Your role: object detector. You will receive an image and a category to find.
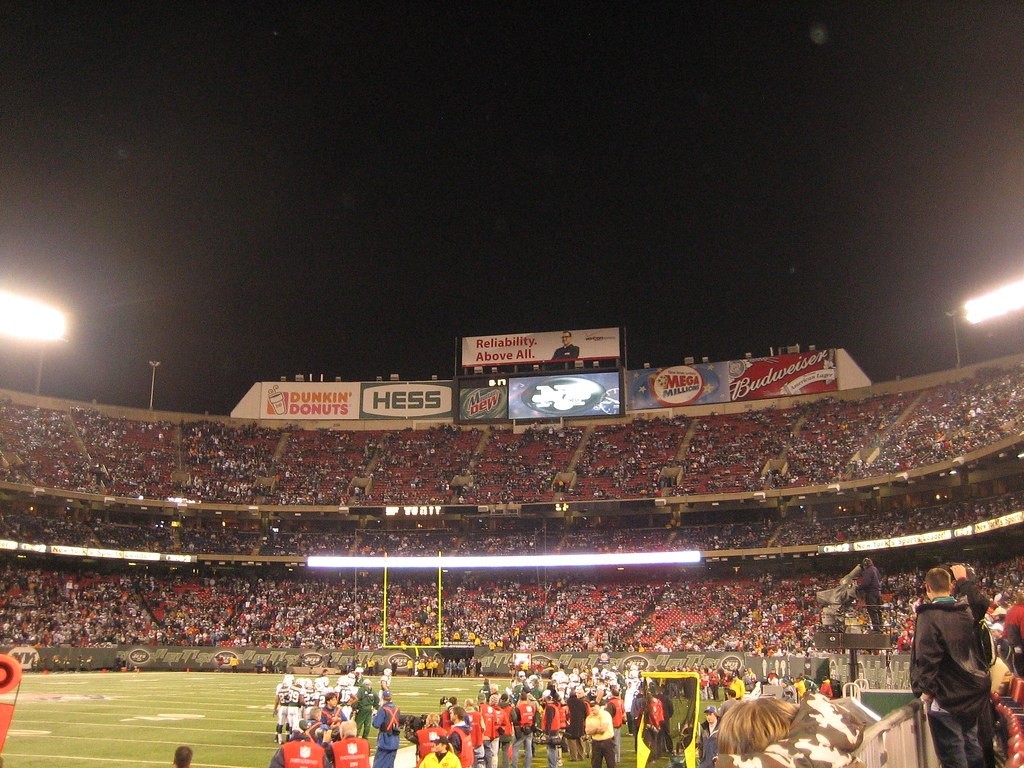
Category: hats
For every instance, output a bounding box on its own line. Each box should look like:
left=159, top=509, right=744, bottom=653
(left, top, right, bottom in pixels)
left=478, top=693, right=486, bottom=703
left=489, top=695, right=498, bottom=705
left=589, top=700, right=598, bottom=704
left=523, top=686, right=532, bottom=693
left=731, top=673, right=739, bottom=678
left=988, top=623, right=1004, bottom=632
left=382, top=690, right=391, bottom=697
left=743, top=668, right=748, bottom=672
left=429, top=735, right=450, bottom=743
left=990, top=606, right=1007, bottom=616
left=501, top=694, right=508, bottom=699
left=531, top=678, right=538, bottom=681
left=703, top=705, right=716, bottom=714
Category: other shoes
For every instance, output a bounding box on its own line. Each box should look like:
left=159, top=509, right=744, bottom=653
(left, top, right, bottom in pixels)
left=451, top=675, right=453, bottom=678
left=461, top=675, right=463, bottom=678
left=438, top=675, right=440, bottom=677
left=392, top=675, right=394, bottom=676
left=408, top=675, right=409, bottom=677
left=556, top=761, right=564, bottom=766
left=432, top=675, right=434, bottom=677
left=367, top=674, right=369, bottom=676
left=232, top=671, right=235, bottom=673
left=577, top=755, right=584, bottom=761
left=435, top=676, right=437, bottom=677
left=395, top=675, right=396, bottom=676
left=869, top=628, right=883, bottom=634
left=236, top=671, right=238, bottom=673
left=458, top=675, right=460, bottom=678
left=625, top=733, right=634, bottom=736
left=440, top=676, right=443, bottom=678
left=410, top=675, right=411, bottom=677
left=419, top=675, right=423, bottom=677
left=427, top=676, right=431, bottom=677
left=569, top=756, right=577, bottom=761
left=377, top=675, right=380, bottom=676
left=454, top=675, right=455, bottom=678
left=375, top=675, right=377, bottom=676
left=370, top=674, right=374, bottom=676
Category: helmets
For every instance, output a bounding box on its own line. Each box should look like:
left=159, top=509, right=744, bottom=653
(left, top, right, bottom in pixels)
left=336, top=676, right=349, bottom=685
left=518, top=671, right=526, bottom=680
left=630, top=665, right=639, bottom=671
left=295, top=678, right=305, bottom=687
left=569, top=674, right=580, bottom=685
left=551, top=672, right=569, bottom=683
left=526, top=675, right=537, bottom=690
left=383, top=668, right=392, bottom=677
left=321, top=677, right=329, bottom=686
left=543, top=690, right=551, bottom=696
left=355, top=667, right=363, bottom=672
left=609, top=678, right=617, bottom=686
left=314, top=678, right=323, bottom=683
left=607, top=672, right=616, bottom=679
left=304, top=678, right=312, bottom=688
left=315, top=683, right=324, bottom=691
left=283, top=675, right=294, bottom=685
left=557, top=691, right=564, bottom=700
left=629, top=671, right=639, bottom=678
left=555, top=684, right=571, bottom=699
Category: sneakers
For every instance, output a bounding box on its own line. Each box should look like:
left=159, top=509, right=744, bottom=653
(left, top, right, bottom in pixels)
left=274, top=735, right=278, bottom=742
left=278, top=735, right=284, bottom=744
left=286, top=734, right=289, bottom=741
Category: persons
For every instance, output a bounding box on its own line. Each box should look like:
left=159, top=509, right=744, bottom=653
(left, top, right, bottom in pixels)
left=552, top=331, right=580, bottom=369
left=0, top=359, right=1024, bottom=768
